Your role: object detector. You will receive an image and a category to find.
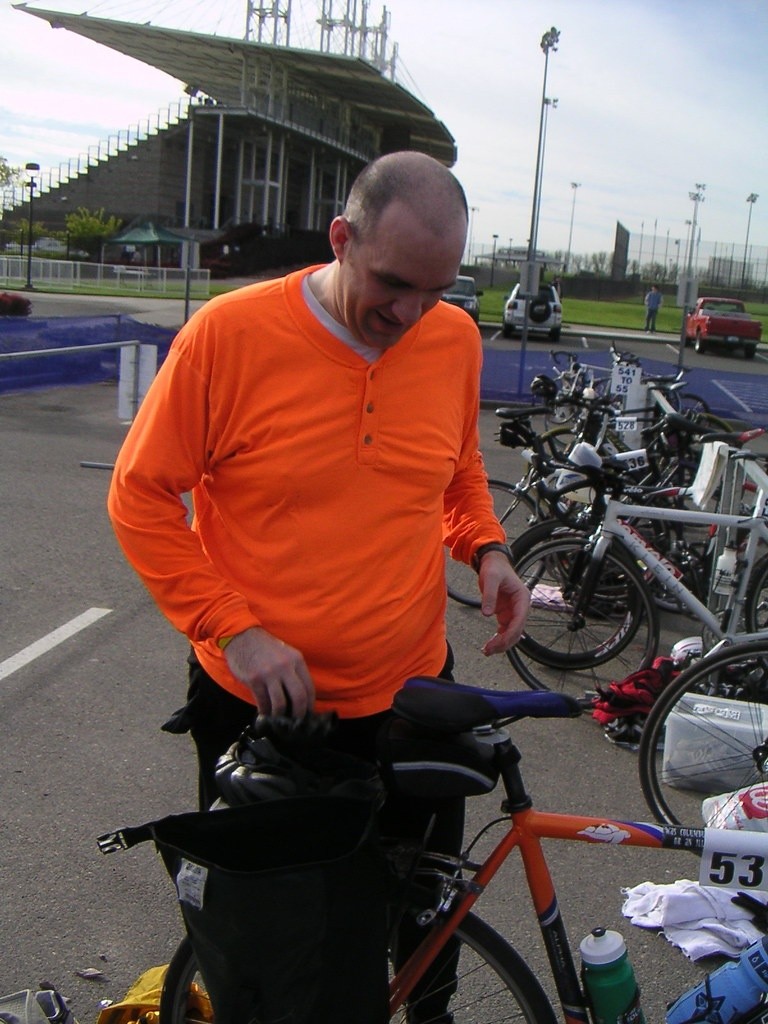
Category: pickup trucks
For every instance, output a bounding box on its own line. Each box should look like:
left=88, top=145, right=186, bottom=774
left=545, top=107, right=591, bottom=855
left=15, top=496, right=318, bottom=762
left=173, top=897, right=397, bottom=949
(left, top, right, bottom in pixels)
left=684, top=298, right=762, bottom=357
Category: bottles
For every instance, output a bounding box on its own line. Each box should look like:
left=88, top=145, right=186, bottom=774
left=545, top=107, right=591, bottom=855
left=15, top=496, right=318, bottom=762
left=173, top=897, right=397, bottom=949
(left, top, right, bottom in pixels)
left=712, top=540, right=737, bottom=596
left=580, top=926, right=648, bottom=1024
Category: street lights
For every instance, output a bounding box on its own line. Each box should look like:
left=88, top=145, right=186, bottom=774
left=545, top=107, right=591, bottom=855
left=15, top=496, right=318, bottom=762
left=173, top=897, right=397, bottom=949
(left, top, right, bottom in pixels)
left=489, top=233, right=499, bottom=289
left=740, top=191, right=760, bottom=289
left=562, top=182, right=582, bottom=271
left=674, top=183, right=707, bottom=280
left=25, top=163, right=43, bottom=289
left=517, top=22, right=560, bottom=397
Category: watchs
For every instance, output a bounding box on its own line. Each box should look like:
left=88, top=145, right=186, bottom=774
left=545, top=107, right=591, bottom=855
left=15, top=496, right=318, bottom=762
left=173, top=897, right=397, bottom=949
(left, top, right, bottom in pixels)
left=471, top=544, right=514, bottom=575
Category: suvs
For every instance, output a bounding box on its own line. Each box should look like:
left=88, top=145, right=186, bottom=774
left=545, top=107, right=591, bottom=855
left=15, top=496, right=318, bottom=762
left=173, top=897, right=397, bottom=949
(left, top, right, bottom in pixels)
left=502, top=282, right=564, bottom=336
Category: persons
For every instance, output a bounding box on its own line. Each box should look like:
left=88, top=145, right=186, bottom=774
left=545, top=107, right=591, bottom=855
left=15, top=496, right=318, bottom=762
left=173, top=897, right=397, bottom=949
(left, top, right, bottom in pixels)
left=105, top=151, right=532, bottom=1024
left=644, top=285, right=663, bottom=334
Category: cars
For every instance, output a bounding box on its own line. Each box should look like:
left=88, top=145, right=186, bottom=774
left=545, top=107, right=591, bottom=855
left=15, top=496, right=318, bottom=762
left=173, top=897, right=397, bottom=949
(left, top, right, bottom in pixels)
left=439, top=275, right=484, bottom=329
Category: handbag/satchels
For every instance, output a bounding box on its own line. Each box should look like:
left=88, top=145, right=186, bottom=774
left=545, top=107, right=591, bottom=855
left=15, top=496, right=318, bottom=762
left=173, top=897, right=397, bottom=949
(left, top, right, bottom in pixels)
left=94, top=794, right=391, bottom=1024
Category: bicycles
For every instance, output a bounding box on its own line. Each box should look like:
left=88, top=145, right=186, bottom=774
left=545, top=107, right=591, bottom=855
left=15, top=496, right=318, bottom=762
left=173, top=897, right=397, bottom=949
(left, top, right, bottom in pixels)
left=93, top=323, right=768, bottom=1024
